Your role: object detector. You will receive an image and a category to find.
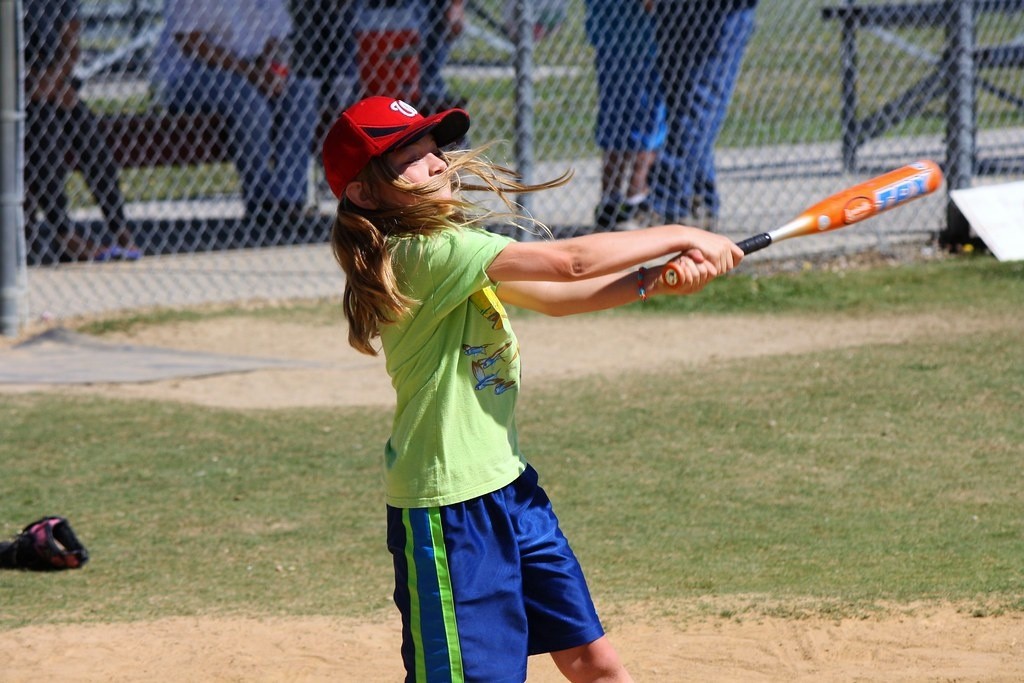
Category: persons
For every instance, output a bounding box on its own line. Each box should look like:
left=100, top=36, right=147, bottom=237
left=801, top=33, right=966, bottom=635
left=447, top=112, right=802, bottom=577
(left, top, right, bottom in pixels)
left=20, top=0, right=142, bottom=261
left=288, top=0, right=470, bottom=152
left=149, top=0, right=332, bottom=241
left=585, top=0, right=662, bottom=230
left=321, top=96, right=744, bottom=683
left=656, top=0, right=757, bottom=231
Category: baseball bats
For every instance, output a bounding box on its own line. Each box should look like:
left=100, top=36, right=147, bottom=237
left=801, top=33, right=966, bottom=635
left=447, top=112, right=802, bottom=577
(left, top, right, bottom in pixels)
left=662, top=159, right=943, bottom=288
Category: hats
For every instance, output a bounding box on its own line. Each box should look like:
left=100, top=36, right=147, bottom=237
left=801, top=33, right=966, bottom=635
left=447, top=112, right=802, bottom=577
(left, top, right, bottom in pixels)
left=321, top=94, right=471, bottom=199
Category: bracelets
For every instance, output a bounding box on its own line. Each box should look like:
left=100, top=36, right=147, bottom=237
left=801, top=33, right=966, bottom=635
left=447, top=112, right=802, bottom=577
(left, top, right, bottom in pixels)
left=638, top=266, right=651, bottom=302
